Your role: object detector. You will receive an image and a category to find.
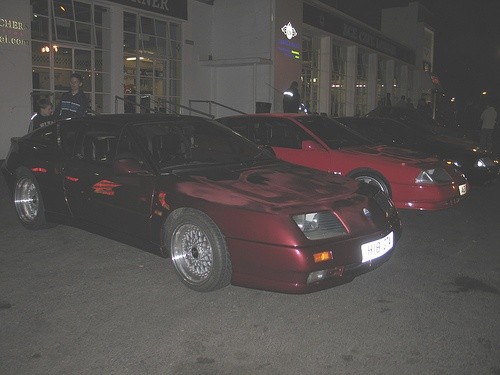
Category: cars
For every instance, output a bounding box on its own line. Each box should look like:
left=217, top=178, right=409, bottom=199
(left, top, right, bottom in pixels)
left=336, top=116, right=500, bottom=188
left=211, top=113, right=471, bottom=230
left=7, top=95, right=404, bottom=292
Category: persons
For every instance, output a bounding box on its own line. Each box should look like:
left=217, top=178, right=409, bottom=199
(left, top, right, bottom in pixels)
left=283, top=80, right=300, bottom=112
left=478, top=99, right=497, bottom=149
left=385, top=92, right=414, bottom=107
left=55, top=73, right=88, bottom=119
left=27, top=97, right=59, bottom=134
